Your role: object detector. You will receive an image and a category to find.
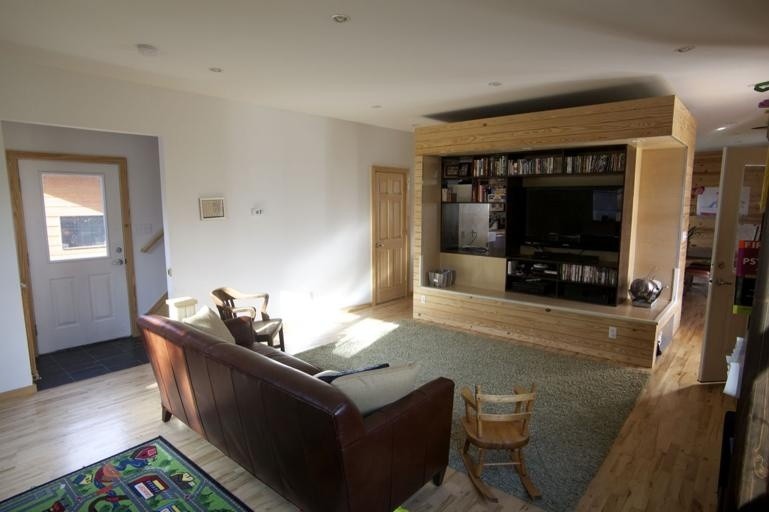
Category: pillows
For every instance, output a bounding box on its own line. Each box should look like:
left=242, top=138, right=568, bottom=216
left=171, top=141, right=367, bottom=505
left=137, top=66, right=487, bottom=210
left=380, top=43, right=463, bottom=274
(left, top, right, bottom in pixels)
left=175, top=301, right=256, bottom=350
left=313, top=358, right=422, bottom=414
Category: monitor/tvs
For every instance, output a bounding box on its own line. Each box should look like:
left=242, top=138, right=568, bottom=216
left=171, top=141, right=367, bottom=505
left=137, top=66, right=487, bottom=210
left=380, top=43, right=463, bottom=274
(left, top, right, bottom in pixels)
left=525, top=184, right=624, bottom=252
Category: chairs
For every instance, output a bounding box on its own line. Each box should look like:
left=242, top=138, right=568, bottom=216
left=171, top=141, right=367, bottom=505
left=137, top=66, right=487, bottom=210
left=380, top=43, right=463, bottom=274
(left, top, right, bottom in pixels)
left=211, top=286, right=285, bottom=353
left=459, top=381, right=545, bottom=502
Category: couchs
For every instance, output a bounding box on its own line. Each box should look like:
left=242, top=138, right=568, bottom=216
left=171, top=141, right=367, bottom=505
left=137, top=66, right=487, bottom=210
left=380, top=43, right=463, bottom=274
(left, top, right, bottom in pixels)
left=137, top=309, right=456, bottom=512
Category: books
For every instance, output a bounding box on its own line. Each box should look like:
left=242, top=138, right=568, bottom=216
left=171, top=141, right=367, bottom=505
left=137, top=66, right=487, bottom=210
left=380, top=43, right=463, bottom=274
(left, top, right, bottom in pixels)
left=471, top=151, right=625, bottom=203
left=560, top=264, right=618, bottom=287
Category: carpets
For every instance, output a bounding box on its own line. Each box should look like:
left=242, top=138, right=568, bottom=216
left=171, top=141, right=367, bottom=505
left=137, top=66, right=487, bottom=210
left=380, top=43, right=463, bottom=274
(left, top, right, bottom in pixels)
left=288, top=315, right=651, bottom=512
left=0, top=433, right=255, bottom=512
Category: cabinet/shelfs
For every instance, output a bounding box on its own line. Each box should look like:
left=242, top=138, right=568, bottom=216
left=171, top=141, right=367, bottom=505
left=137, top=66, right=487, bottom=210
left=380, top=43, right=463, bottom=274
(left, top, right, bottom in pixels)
left=437, top=145, right=642, bottom=309
